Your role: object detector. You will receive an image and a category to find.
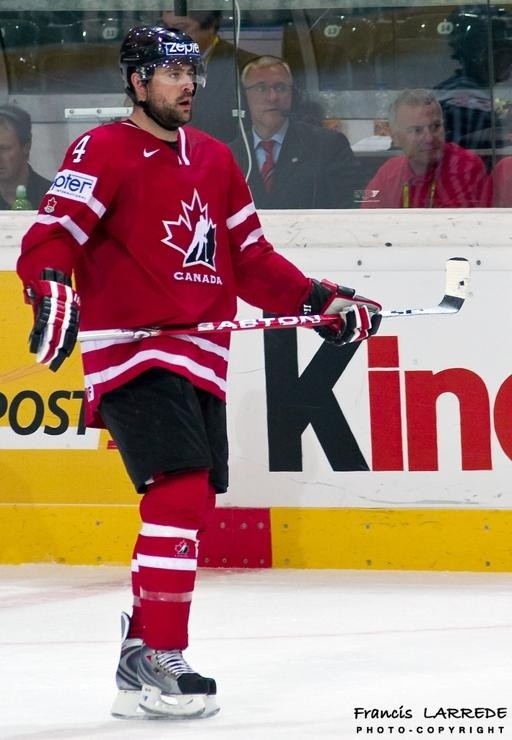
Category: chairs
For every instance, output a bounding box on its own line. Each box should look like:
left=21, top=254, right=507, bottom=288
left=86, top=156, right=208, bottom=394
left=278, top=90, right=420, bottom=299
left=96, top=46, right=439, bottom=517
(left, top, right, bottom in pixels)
left=478, top=10, right=512, bottom=88
left=282, top=15, right=396, bottom=89
left=42, top=23, right=81, bottom=44
left=0, top=19, right=40, bottom=47
left=393, top=9, right=483, bottom=90
left=79, top=18, right=143, bottom=42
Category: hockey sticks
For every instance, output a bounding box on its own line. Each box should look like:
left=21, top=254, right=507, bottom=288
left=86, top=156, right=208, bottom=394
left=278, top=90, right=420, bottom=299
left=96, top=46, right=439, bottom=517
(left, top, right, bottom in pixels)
left=76, top=256, right=472, bottom=344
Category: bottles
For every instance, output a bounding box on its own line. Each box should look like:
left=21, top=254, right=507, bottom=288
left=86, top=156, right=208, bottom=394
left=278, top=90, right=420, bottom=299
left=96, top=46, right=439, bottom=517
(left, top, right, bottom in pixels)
left=10, top=184, right=33, bottom=211
left=374, top=82, right=393, bottom=137
left=321, top=85, right=340, bottom=131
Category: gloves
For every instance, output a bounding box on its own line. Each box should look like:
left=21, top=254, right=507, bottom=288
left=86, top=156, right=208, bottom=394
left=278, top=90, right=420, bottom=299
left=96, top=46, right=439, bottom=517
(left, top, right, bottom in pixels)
left=301, top=279, right=383, bottom=345
left=27, top=269, right=81, bottom=372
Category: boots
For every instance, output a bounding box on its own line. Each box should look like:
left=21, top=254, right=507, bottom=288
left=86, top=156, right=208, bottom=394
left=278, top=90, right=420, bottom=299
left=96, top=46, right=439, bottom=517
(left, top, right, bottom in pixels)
left=115, top=612, right=216, bottom=694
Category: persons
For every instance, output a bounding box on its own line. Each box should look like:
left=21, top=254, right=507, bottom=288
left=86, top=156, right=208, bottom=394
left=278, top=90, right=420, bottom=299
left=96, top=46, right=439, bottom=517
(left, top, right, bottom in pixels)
left=0, top=105, right=54, bottom=213
left=355, top=88, right=486, bottom=207
left=13, top=20, right=383, bottom=700
left=158, top=8, right=262, bottom=150
left=227, top=55, right=359, bottom=207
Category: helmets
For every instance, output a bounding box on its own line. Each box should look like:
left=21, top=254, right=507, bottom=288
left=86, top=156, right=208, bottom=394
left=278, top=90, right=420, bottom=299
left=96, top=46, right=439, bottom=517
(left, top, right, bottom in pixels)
left=120, top=24, right=201, bottom=89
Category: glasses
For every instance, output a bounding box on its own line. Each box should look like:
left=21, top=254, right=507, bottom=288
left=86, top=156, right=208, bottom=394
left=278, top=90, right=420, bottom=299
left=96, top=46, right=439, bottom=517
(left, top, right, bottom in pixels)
left=245, top=83, right=288, bottom=95
